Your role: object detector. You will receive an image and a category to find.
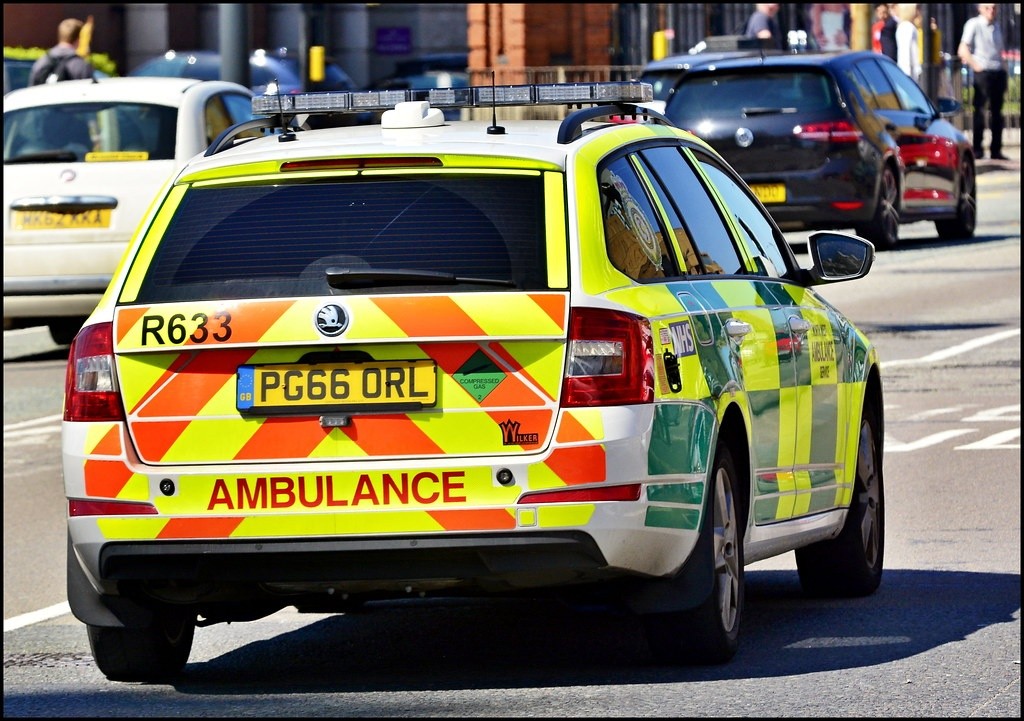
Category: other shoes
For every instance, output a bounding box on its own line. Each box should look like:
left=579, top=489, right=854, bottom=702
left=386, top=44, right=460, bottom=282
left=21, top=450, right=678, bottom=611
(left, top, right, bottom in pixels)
left=991, top=155, right=1007, bottom=159
left=974, top=155, right=981, bottom=159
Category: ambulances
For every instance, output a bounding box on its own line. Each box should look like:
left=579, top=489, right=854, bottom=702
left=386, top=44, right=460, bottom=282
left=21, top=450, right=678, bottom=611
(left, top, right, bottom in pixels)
left=60, top=81, right=885, bottom=680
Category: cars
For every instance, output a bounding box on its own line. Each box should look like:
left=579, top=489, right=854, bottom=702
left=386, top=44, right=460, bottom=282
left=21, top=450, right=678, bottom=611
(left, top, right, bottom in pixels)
left=0, top=39, right=471, bottom=346
left=631, top=49, right=977, bottom=247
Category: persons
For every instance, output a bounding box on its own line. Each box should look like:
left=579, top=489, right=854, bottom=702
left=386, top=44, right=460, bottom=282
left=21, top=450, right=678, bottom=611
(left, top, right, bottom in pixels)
left=27, top=18, right=91, bottom=87
left=957, top=2, right=1024, bottom=160
left=809, top=3, right=942, bottom=96
left=743, top=3, right=784, bottom=55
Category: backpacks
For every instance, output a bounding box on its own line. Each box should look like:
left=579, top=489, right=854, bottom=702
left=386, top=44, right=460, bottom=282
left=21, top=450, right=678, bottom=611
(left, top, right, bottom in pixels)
left=33, top=50, right=78, bottom=85
